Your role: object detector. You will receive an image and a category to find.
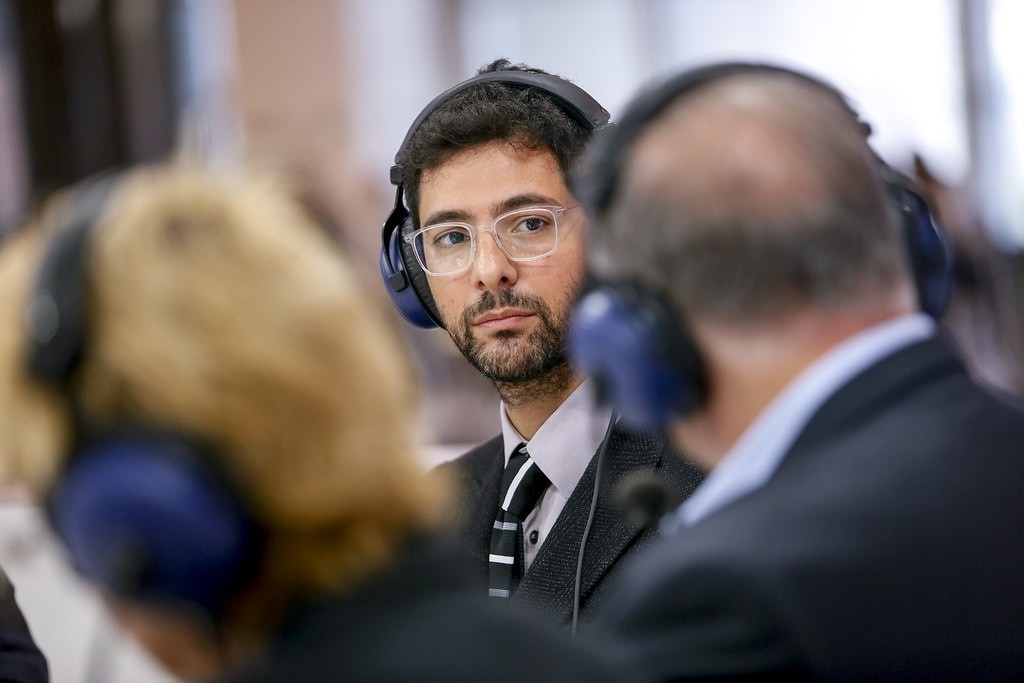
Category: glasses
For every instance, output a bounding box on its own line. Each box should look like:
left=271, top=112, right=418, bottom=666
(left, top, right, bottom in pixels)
left=403, top=201, right=580, bottom=276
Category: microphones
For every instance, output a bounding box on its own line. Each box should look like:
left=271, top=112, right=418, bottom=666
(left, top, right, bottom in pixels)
left=618, top=441, right=671, bottom=513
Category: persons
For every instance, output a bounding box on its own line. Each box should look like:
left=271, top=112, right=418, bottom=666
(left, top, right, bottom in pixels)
left=381, top=58, right=703, bottom=633
left=0, top=165, right=618, bottom=682
left=570, top=59, right=1023, bottom=683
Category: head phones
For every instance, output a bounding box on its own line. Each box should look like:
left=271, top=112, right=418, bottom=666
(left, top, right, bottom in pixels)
left=28, top=167, right=268, bottom=620
left=379, top=71, right=608, bottom=332
left=564, top=60, right=958, bottom=433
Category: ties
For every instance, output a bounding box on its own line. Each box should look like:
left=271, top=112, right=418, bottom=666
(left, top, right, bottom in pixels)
left=486, top=451, right=549, bottom=607
